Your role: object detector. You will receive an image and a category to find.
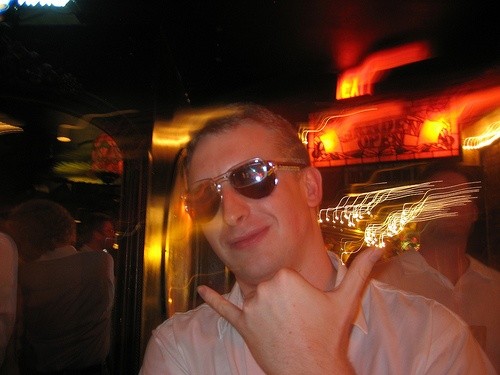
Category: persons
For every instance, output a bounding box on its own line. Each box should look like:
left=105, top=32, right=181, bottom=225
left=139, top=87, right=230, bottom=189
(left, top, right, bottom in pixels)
left=364, top=169, right=500, bottom=374
left=138, top=104, right=497, bottom=375
left=80, top=212, right=115, bottom=256
left=19, top=198, right=115, bottom=375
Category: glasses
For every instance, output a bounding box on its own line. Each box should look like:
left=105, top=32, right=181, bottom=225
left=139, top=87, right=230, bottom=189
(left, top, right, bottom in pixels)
left=181, top=158, right=312, bottom=224
